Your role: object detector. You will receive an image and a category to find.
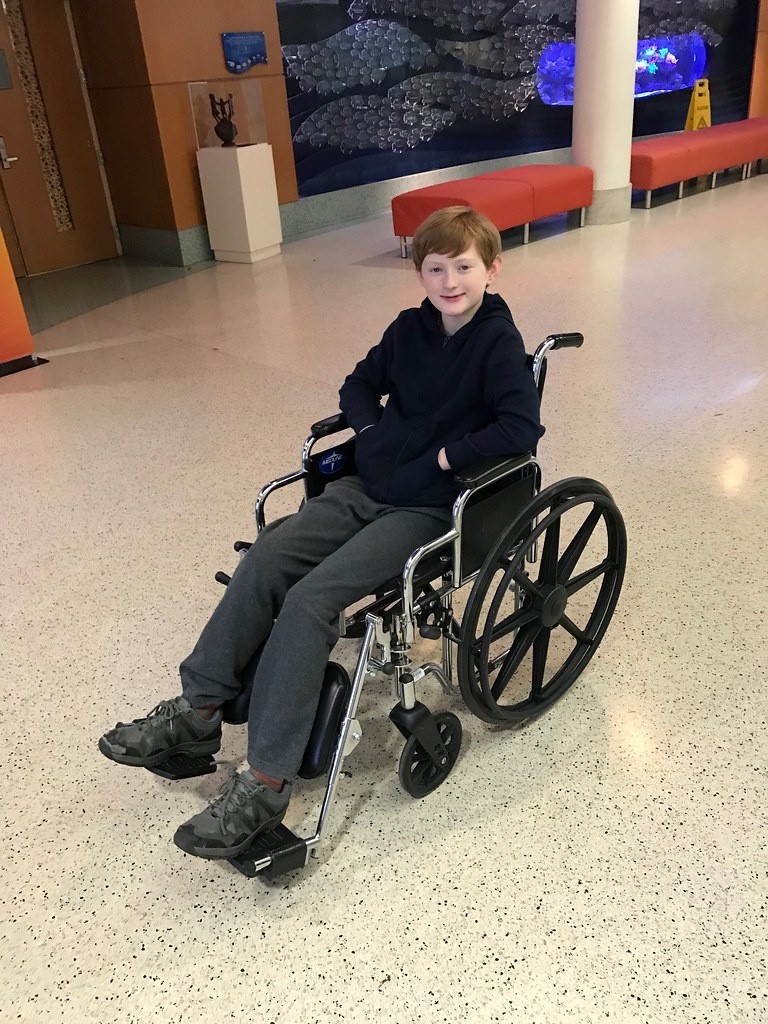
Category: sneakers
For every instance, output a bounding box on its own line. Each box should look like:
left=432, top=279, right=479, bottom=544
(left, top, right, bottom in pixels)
left=98, top=696, right=223, bottom=767
left=174, top=760, right=294, bottom=861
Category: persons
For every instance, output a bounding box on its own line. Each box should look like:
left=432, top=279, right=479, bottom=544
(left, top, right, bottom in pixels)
left=95, top=201, right=547, bottom=862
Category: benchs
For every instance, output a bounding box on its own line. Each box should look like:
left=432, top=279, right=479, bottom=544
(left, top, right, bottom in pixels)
left=739, top=117, right=768, bottom=178
left=391, top=163, right=593, bottom=259
left=631, top=122, right=756, bottom=209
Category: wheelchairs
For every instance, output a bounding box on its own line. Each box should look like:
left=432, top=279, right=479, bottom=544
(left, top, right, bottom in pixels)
left=146, top=328, right=628, bottom=883
